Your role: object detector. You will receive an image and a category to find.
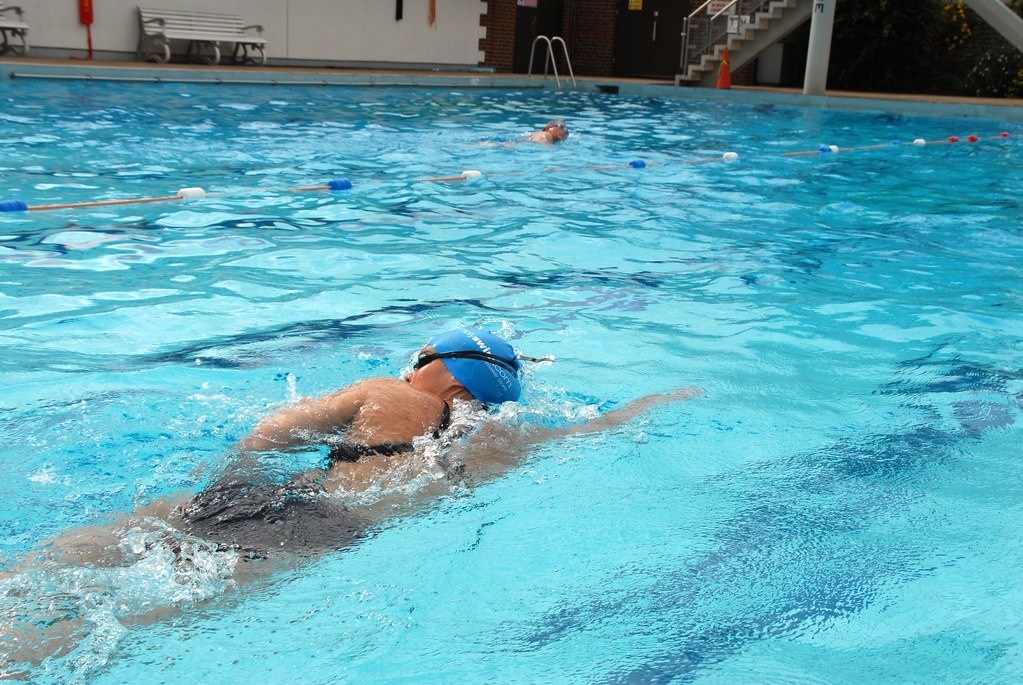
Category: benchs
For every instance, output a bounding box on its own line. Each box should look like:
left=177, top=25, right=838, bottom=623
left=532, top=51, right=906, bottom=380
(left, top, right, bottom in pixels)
left=137, top=4, right=269, bottom=66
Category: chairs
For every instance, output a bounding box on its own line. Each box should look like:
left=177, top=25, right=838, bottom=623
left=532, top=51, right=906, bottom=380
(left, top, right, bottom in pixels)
left=0, top=2, right=32, bottom=58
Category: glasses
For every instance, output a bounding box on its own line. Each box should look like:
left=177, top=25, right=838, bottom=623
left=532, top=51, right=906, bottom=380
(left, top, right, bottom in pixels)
left=413, top=351, right=521, bottom=375
left=549, top=123, right=569, bottom=136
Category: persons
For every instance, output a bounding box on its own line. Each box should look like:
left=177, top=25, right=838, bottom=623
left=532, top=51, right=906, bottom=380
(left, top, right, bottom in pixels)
left=480, top=121, right=570, bottom=152
left=2, top=323, right=701, bottom=672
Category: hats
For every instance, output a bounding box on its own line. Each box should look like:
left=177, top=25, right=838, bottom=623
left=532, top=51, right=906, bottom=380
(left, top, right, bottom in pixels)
left=425, top=329, right=520, bottom=410
